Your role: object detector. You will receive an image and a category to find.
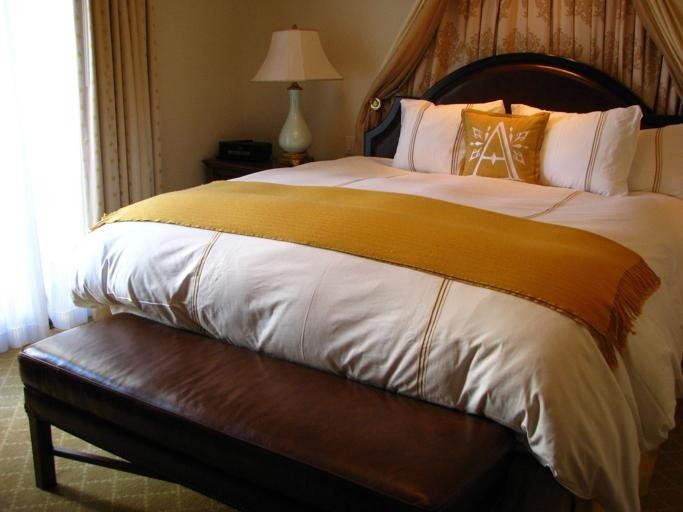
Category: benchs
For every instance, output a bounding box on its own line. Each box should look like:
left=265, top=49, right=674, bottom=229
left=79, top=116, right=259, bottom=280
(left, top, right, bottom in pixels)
left=19, top=313, right=526, bottom=512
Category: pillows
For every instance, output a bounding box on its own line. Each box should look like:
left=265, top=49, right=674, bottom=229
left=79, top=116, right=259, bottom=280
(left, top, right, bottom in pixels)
left=391, top=95, right=681, bottom=198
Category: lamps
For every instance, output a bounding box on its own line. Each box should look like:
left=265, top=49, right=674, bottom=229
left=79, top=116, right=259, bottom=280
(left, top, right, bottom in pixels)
left=249, top=22, right=344, bottom=167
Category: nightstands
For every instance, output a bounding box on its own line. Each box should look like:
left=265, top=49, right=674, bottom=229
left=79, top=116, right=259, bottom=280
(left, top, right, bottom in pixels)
left=210, top=158, right=276, bottom=180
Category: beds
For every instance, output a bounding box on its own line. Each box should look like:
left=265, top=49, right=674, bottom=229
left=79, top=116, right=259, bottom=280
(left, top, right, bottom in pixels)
left=98, top=51, right=682, bottom=511
